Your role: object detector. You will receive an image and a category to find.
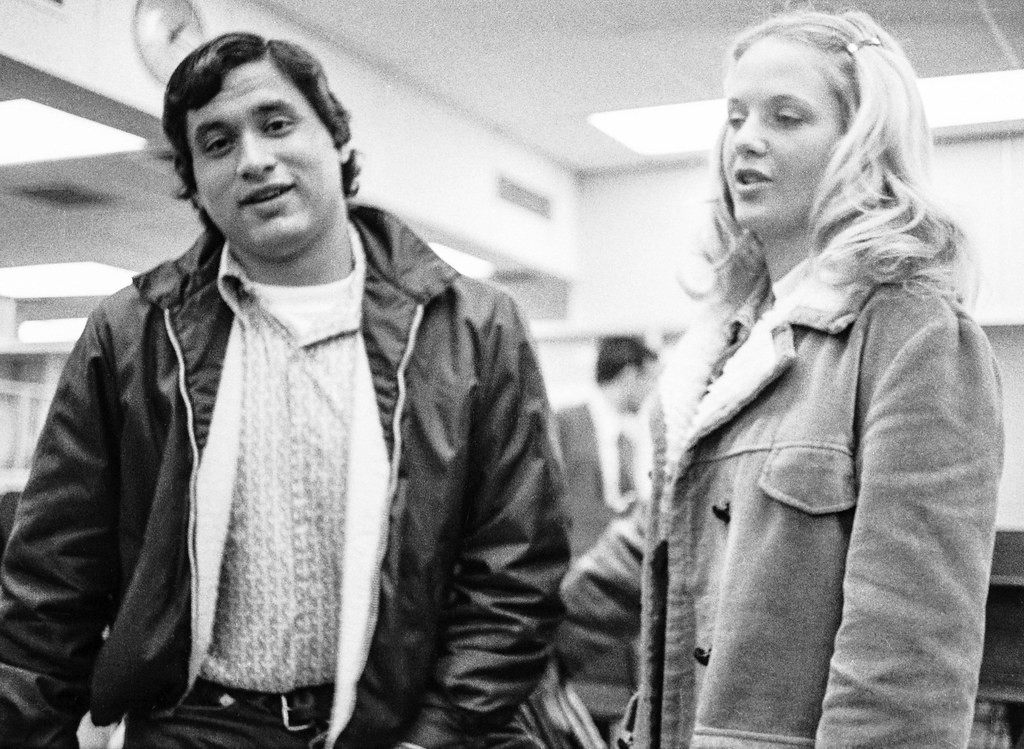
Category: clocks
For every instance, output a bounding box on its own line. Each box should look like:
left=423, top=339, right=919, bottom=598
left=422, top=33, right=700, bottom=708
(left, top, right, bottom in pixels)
left=96, top=0, right=217, bottom=102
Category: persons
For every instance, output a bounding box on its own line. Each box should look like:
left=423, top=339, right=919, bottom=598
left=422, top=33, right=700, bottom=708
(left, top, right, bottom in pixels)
left=556, top=332, right=660, bottom=748
left=0, top=32, right=572, bottom=749
left=529, top=7, right=1008, bottom=749
